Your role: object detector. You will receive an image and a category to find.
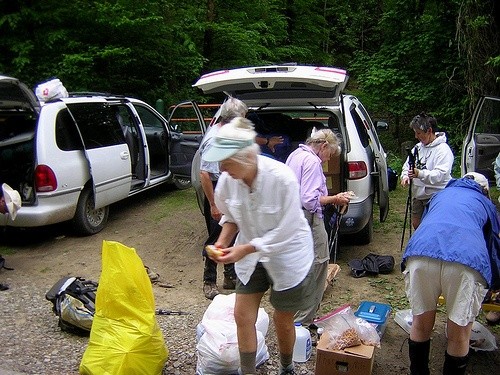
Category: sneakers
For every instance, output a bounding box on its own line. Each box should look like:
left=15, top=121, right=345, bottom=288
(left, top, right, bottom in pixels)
left=223, top=277, right=237, bottom=289
left=203, top=280, right=219, bottom=299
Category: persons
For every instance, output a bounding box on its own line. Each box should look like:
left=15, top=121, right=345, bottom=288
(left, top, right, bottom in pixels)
left=199, top=97, right=283, bottom=299
left=0, top=183, right=21, bottom=290
left=402, top=112, right=454, bottom=229
left=204, top=118, right=315, bottom=375
left=400, top=171, right=500, bottom=375
left=285, top=128, right=349, bottom=346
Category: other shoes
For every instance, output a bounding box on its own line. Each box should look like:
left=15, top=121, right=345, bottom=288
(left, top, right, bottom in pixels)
left=278, top=362, right=295, bottom=375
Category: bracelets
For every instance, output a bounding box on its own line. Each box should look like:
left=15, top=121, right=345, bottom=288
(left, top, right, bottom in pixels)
left=265, top=138, right=270, bottom=145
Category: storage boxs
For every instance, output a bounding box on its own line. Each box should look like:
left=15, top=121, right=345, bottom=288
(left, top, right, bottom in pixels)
left=324, top=174, right=341, bottom=194
left=355, top=301, right=391, bottom=340
left=315, top=334, right=374, bottom=375
left=322, top=154, right=341, bottom=173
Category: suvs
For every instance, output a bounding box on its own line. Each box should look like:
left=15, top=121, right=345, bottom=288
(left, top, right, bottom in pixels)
left=191, top=63, right=391, bottom=246
left=0, top=75, right=207, bottom=237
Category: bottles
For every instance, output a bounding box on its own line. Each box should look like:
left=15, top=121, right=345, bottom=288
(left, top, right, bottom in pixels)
left=292, top=322, right=312, bottom=363
left=317, top=327, right=323, bottom=347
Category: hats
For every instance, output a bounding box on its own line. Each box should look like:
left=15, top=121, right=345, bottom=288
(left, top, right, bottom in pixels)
left=202, top=137, right=253, bottom=161
left=465, top=171, right=491, bottom=199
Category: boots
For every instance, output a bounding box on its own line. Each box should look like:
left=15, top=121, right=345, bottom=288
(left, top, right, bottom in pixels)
left=406, top=335, right=429, bottom=375
left=444, top=351, right=471, bottom=375
